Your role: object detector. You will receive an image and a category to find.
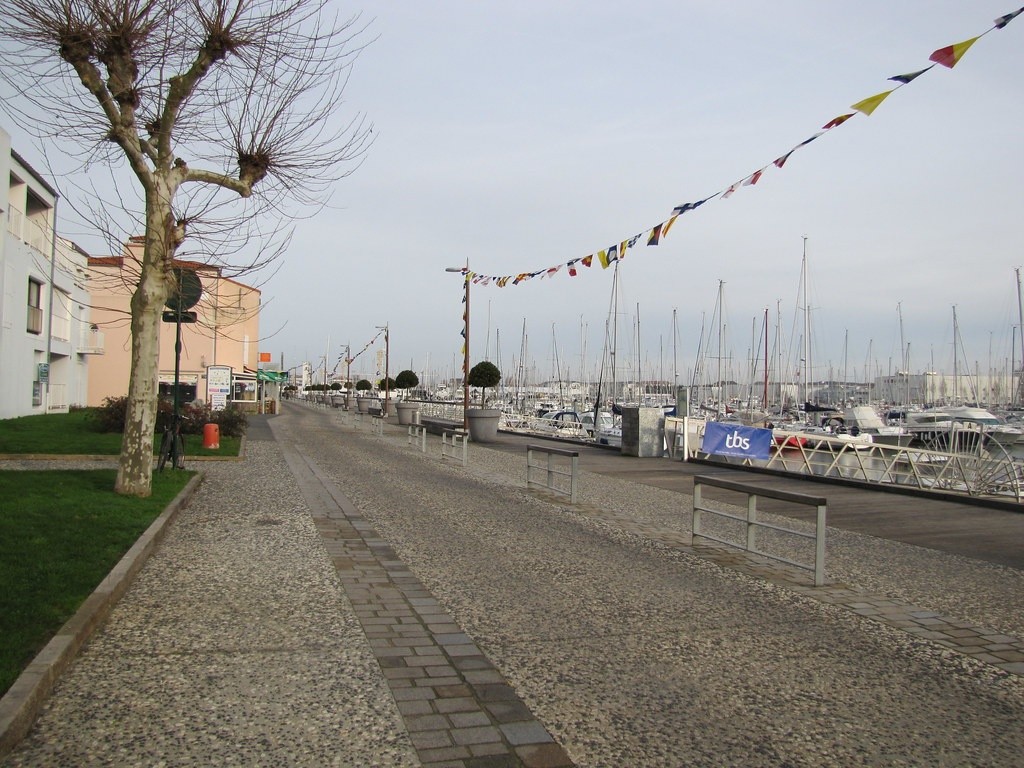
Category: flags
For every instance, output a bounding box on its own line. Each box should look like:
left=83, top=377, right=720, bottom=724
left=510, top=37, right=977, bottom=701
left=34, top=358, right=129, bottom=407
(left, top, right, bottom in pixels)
left=461, top=266, right=470, bottom=389
left=662, top=215, right=679, bottom=238
left=626, top=235, right=642, bottom=248
left=669, top=4, right=1024, bottom=216
left=647, top=223, right=664, bottom=246
left=620, top=240, right=628, bottom=258
left=596, top=250, right=610, bottom=270
left=470, top=253, right=581, bottom=288
left=606, top=245, right=618, bottom=264
left=581, top=255, right=593, bottom=268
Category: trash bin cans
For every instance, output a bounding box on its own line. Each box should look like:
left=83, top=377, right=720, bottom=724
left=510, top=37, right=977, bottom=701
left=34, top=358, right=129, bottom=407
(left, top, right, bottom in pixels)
left=202, top=422, right=221, bottom=450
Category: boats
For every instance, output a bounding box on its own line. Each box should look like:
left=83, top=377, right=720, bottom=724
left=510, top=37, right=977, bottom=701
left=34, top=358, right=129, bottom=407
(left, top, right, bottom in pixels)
left=377, top=236, right=1023, bottom=451
